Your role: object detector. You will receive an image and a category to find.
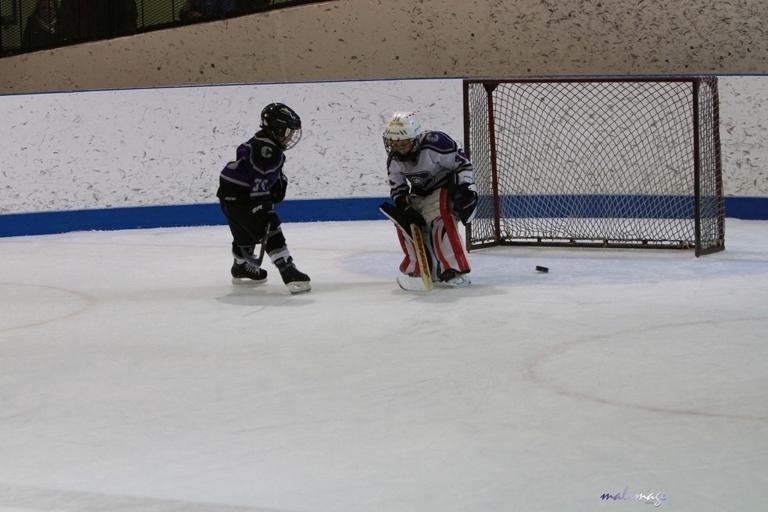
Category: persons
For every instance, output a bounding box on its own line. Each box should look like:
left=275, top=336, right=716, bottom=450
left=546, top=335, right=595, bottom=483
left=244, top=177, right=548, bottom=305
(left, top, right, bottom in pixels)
left=216, top=102, right=311, bottom=286
left=380, top=110, right=479, bottom=283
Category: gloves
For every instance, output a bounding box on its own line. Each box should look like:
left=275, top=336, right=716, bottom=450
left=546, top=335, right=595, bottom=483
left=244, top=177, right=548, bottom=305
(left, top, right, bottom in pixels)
left=269, top=178, right=287, bottom=204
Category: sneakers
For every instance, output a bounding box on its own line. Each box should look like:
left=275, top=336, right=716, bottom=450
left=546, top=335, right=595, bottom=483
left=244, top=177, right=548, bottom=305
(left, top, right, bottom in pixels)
left=274, top=256, right=310, bottom=284
left=231, top=258, right=267, bottom=280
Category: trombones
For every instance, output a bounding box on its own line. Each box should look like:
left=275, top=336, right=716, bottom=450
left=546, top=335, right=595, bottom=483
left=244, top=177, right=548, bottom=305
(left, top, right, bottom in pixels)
left=396, top=195, right=433, bottom=292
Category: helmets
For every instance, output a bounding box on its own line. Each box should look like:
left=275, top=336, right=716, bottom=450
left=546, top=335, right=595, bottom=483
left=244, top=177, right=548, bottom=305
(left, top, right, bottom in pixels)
left=260, top=103, right=302, bottom=150
left=383, top=112, right=425, bottom=162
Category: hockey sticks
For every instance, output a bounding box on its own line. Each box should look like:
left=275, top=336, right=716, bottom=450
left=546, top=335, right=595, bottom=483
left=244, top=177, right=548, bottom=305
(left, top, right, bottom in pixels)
left=242, top=202, right=275, bottom=269
